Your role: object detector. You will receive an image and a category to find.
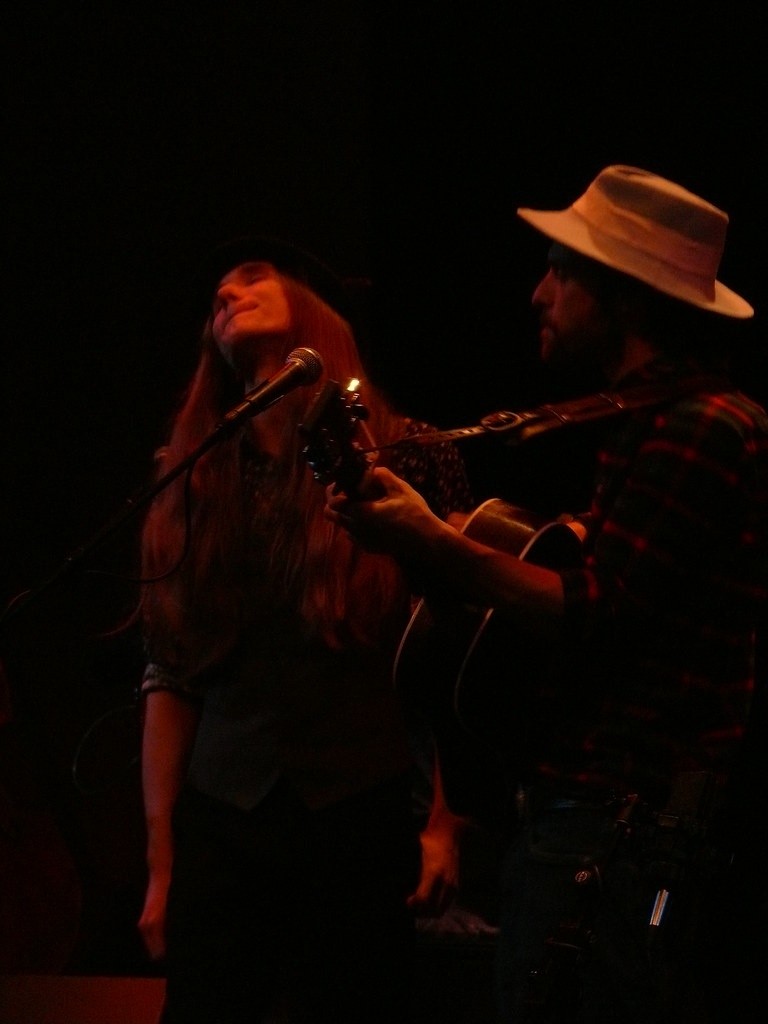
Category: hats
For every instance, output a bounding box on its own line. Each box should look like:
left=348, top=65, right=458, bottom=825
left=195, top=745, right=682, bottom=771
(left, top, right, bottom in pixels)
left=194, top=237, right=348, bottom=316
left=518, top=165, right=755, bottom=320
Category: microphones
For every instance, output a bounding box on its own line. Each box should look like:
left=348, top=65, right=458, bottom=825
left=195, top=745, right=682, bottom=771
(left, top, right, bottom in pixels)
left=217, top=346, right=322, bottom=436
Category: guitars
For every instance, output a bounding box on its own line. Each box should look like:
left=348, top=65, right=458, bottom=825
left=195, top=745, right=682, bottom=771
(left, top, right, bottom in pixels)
left=300, top=381, right=601, bottom=765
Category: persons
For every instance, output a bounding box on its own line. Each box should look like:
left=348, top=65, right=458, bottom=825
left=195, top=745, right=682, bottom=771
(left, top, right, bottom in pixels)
left=137, top=260, right=461, bottom=1024
left=324, top=164, right=768, bottom=1024
